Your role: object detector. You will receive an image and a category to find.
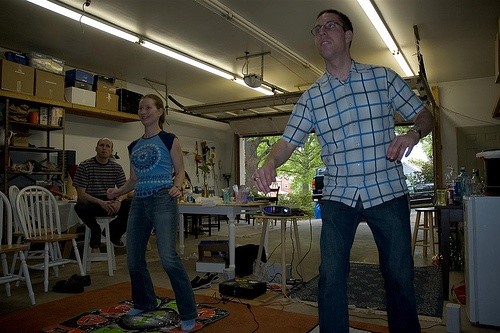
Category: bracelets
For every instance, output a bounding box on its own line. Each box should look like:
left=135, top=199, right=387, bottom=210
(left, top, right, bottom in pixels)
left=176, top=186, right=181, bottom=190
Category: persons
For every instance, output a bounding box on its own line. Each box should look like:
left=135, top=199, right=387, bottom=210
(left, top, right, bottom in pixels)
left=107, top=94, right=198, bottom=331
left=72, top=137, right=132, bottom=249
left=254, top=9, right=436, bottom=333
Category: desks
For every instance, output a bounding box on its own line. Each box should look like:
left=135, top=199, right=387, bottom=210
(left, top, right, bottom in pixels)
left=176, top=200, right=270, bottom=280
left=436, top=204, right=465, bottom=273
left=253, top=215, right=308, bottom=296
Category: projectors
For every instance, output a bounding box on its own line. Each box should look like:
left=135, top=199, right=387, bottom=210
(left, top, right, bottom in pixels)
left=263, top=205, right=302, bottom=217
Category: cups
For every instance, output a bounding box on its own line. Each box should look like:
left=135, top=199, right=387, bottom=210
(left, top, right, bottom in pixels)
left=29, top=111, right=39, bottom=124
left=437, top=190, right=448, bottom=206
left=235, top=192, right=248, bottom=204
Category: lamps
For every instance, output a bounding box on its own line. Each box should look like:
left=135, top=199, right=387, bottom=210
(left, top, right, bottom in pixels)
left=356, top=0, right=414, bottom=77
left=28, top=0, right=325, bottom=95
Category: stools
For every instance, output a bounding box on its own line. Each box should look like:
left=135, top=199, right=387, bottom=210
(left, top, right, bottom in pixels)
left=411, top=207, right=439, bottom=262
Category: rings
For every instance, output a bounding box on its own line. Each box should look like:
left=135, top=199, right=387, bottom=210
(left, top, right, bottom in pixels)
left=254, top=177, right=260, bottom=181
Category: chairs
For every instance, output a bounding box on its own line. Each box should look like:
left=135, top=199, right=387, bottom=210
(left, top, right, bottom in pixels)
left=0, top=183, right=281, bottom=308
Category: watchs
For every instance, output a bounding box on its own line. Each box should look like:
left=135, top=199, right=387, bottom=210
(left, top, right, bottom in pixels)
left=411, top=128, right=422, bottom=139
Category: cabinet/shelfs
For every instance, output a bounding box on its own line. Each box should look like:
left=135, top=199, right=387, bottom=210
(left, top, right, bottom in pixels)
left=0, top=98, right=66, bottom=199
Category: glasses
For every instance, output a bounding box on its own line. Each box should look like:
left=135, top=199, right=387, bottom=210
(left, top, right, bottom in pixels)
left=311, top=20, right=345, bottom=37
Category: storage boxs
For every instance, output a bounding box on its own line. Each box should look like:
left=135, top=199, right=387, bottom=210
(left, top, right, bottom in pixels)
left=29, top=111, right=40, bottom=124
left=0, top=51, right=30, bottom=66
left=51, top=107, right=64, bottom=127
left=195, top=239, right=230, bottom=274
left=65, top=69, right=94, bottom=85
left=97, top=82, right=117, bottom=94
left=64, top=86, right=97, bottom=108
left=0, top=60, right=34, bottom=96
left=40, top=107, right=48, bottom=125
left=94, top=75, right=114, bottom=85
left=96, top=91, right=119, bottom=112
left=35, top=69, right=66, bottom=103
left=12, top=131, right=33, bottom=147
left=115, top=88, right=145, bottom=115
left=28, top=51, right=65, bottom=76
left=64, top=80, right=93, bottom=91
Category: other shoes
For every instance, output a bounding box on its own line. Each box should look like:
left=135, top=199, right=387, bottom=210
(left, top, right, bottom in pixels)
left=101, top=229, right=124, bottom=247
left=67, top=274, right=91, bottom=287
left=89, top=228, right=101, bottom=248
left=52, top=279, right=85, bottom=294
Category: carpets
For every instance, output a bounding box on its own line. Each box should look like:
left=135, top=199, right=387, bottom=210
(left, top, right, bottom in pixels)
left=0, top=280, right=390, bottom=333
left=285, top=262, right=451, bottom=322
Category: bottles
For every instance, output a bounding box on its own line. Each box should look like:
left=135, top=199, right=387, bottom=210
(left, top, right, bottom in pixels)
left=248, top=191, right=255, bottom=201
left=447, top=168, right=479, bottom=204
left=185, top=185, right=193, bottom=201
